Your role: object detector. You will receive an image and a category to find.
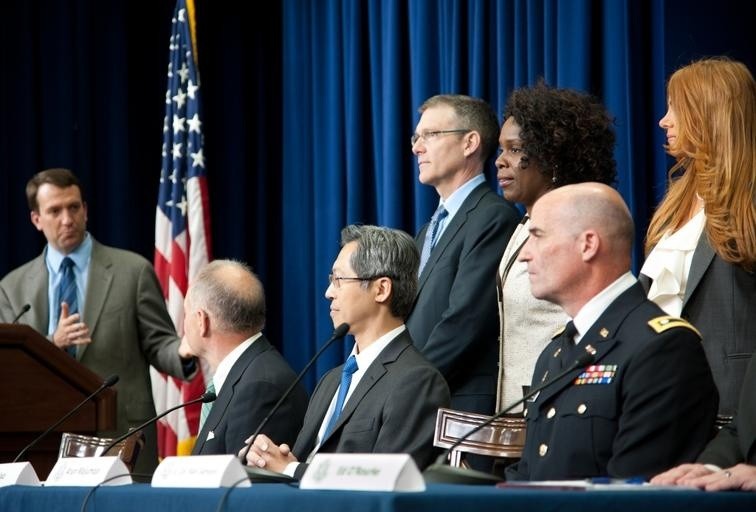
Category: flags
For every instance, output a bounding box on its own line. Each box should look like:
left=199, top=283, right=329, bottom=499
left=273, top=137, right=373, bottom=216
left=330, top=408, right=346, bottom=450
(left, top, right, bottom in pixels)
left=153, top=1, right=213, bottom=459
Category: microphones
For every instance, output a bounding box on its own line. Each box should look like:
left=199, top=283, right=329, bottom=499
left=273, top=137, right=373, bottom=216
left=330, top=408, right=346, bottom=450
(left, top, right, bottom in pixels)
left=421, top=351, right=592, bottom=484
left=100, top=393, right=216, bottom=456
left=238, top=323, right=350, bottom=482
left=13, top=373, right=120, bottom=462
left=12, top=304, right=30, bottom=323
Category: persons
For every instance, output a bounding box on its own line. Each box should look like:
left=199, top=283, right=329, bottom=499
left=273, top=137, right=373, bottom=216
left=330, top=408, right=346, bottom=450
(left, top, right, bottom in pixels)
left=638, top=56, right=756, bottom=430
left=502, top=181, right=720, bottom=478
left=495, top=75, right=619, bottom=408
left=404, top=92, right=523, bottom=473
left=0, top=165, right=200, bottom=474
left=182, top=257, right=308, bottom=454
left=649, top=352, right=756, bottom=491
left=239, top=219, right=451, bottom=482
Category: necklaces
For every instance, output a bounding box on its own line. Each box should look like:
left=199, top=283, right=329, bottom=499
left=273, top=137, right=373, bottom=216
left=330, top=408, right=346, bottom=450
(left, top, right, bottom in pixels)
left=696, top=192, right=705, bottom=201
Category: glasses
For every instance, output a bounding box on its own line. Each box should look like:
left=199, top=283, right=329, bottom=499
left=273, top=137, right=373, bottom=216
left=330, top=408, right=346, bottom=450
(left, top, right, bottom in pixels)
left=410, top=127, right=470, bottom=145
left=327, top=272, right=389, bottom=288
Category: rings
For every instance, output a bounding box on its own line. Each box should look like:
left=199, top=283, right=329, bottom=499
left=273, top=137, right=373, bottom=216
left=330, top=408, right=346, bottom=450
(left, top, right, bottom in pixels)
left=722, top=470, right=733, bottom=478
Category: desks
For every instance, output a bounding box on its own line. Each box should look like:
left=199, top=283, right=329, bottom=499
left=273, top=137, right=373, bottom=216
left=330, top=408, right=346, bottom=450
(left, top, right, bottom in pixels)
left=0, top=483, right=756, bottom=512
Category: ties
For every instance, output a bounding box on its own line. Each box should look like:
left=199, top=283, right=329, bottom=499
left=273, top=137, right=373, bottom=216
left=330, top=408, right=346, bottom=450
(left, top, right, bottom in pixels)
left=561, top=319, right=578, bottom=368
left=429, top=204, right=448, bottom=256
left=53, top=255, right=78, bottom=361
left=320, top=354, right=360, bottom=450
left=195, top=380, right=216, bottom=440
